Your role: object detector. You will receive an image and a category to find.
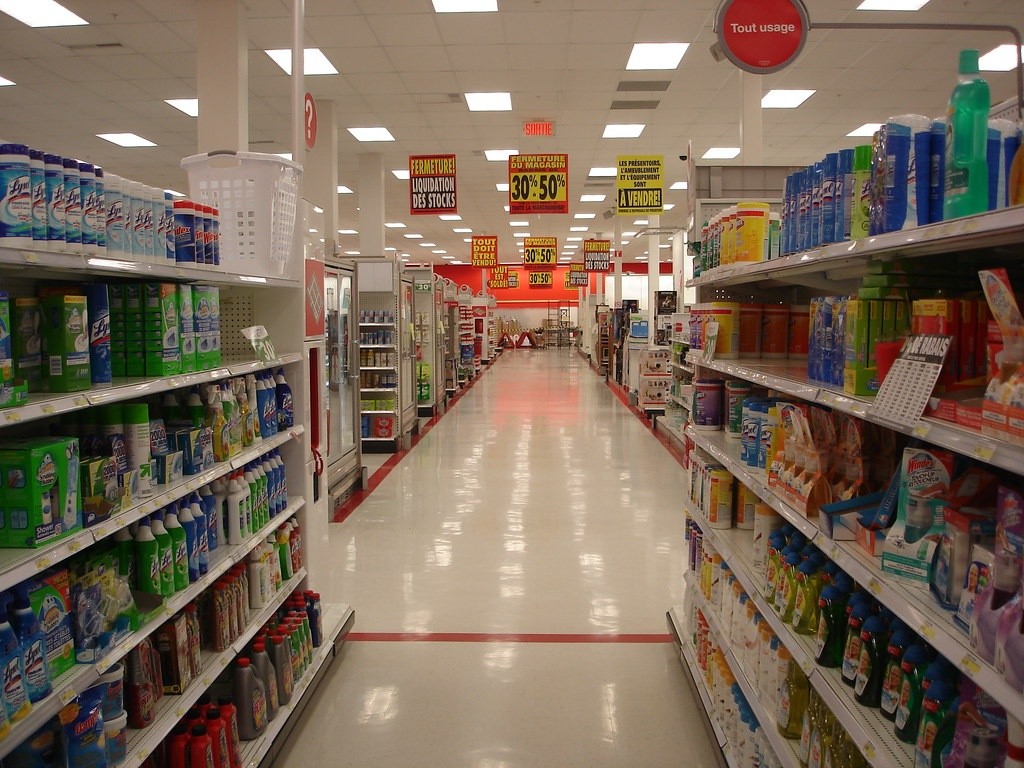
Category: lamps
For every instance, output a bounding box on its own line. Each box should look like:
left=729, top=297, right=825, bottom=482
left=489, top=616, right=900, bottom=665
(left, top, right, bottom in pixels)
left=603, top=210, right=615, bottom=220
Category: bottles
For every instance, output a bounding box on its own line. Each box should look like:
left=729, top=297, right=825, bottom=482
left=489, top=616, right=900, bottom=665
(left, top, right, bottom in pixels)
left=360, top=349, right=375, bottom=367
left=262, top=589, right=324, bottom=680
left=361, top=371, right=366, bottom=388
left=366, top=371, right=371, bottom=388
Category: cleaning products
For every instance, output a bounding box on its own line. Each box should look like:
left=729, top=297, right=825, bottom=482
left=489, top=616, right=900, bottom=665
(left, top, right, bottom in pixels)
left=0, top=139, right=321, bottom=768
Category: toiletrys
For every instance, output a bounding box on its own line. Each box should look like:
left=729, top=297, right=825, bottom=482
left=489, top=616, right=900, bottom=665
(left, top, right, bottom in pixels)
left=677, top=46, right=1024, bottom=768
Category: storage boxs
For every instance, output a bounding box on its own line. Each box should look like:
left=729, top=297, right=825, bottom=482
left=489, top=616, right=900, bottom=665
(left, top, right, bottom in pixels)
left=0, top=419, right=214, bottom=551
left=542, top=319, right=556, bottom=340
left=839, top=260, right=1024, bottom=448
left=181, top=150, right=304, bottom=276
left=499, top=317, right=524, bottom=341
left=0, top=279, right=223, bottom=410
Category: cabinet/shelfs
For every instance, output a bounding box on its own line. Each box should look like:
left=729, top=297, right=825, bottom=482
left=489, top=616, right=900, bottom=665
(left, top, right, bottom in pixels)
left=445, top=278, right=499, bottom=398
left=666, top=46, right=1024, bottom=768
left=0, top=145, right=353, bottom=768
left=405, top=262, right=449, bottom=416
left=359, top=251, right=422, bottom=455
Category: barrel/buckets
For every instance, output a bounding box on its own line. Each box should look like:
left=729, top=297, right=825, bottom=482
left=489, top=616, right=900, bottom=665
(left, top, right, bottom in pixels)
left=165, top=627, right=294, bottom=768
